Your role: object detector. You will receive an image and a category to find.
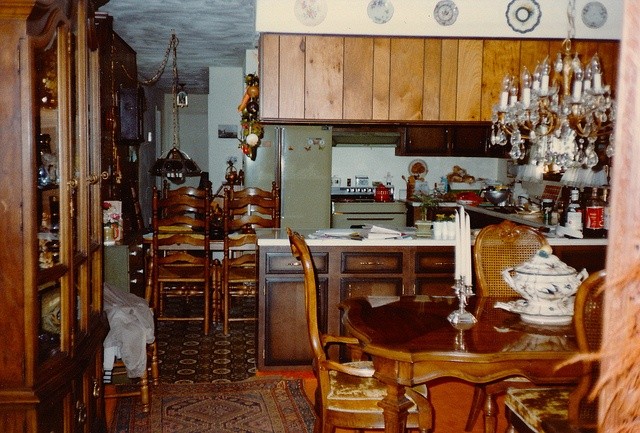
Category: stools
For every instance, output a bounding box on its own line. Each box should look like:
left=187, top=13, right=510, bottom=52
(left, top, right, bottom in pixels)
left=104, top=341, right=159, bottom=412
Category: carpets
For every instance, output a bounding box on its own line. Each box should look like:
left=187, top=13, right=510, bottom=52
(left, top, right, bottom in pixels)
left=129, top=377, right=317, bottom=432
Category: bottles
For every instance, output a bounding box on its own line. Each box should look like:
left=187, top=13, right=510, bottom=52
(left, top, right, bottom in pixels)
left=602, top=187, right=609, bottom=238
left=584, top=187, right=604, bottom=237
left=43, top=194, right=60, bottom=263
left=566, top=188, right=582, bottom=226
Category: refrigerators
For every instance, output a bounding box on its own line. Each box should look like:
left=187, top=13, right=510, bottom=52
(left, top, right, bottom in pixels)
left=244, top=122, right=332, bottom=229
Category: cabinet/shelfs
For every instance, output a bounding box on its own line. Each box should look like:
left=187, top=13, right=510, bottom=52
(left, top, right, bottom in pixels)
left=90, top=25, right=109, bottom=333
left=451, top=127, right=493, bottom=156
left=490, top=124, right=532, bottom=155
left=101, top=29, right=145, bottom=295
left=2, top=5, right=87, bottom=387
left=400, top=126, right=450, bottom=157
left=1, top=365, right=88, bottom=432
left=88, top=335, right=107, bottom=433
left=259, top=31, right=619, bottom=126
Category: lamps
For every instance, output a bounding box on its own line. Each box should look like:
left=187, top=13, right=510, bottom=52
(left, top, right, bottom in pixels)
left=304, top=137, right=326, bottom=152
left=490, top=1, right=616, bottom=169
left=152, top=28, right=202, bottom=184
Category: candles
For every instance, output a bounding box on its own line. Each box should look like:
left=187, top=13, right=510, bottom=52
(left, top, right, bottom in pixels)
left=454, top=205, right=474, bottom=286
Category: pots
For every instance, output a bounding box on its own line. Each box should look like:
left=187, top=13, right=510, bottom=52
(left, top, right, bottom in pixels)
left=483, top=190, right=509, bottom=204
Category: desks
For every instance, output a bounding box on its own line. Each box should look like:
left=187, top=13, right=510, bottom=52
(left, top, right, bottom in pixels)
left=258, top=230, right=604, bottom=375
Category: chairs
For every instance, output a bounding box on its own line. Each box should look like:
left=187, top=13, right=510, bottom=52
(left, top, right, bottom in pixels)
left=159, top=179, right=212, bottom=317
left=229, top=181, right=276, bottom=301
left=503, top=269, right=605, bottom=432
left=223, top=187, right=280, bottom=336
left=153, top=187, right=209, bottom=336
left=465, top=221, right=548, bottom=433
left=286, top=227, right=433, bottom=433
